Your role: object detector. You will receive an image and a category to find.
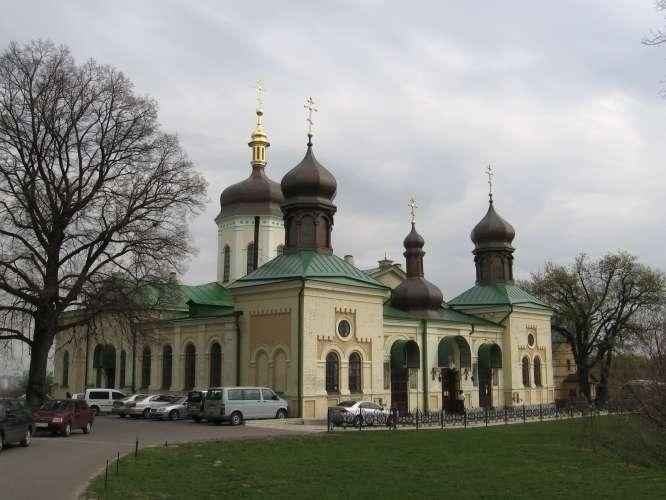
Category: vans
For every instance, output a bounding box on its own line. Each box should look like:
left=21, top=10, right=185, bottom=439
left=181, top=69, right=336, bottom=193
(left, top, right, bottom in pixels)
left=72, top=389, right=128, bottom=416
left=199, top=386, right=289, bottom=426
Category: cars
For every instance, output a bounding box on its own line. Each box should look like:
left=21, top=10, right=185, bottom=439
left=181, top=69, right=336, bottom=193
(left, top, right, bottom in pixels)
left=328, top=400, right=399, bottom=427
left=0, top=394, right=94, bottom=452
left=112, top=391, right=222, bottom=423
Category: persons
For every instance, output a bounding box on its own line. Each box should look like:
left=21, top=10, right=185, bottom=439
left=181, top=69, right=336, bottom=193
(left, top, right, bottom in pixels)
left=457, top=391, right=466, bottom=415
